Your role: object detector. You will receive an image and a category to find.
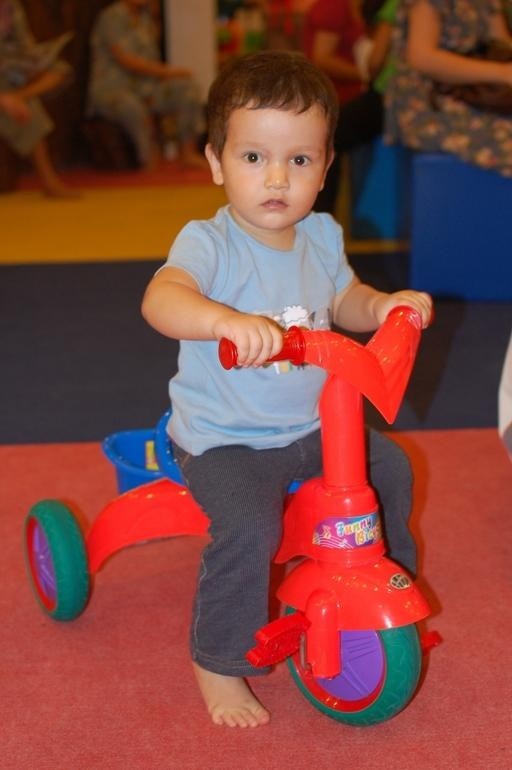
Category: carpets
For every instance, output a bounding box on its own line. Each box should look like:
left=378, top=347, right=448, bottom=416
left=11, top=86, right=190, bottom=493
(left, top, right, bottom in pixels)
left=2, top=426, right=512, bottom=768
left=1, top=161, right=415, bottom=268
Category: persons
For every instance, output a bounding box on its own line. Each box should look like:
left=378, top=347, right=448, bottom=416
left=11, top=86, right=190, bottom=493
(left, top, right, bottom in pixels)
left=2, top=3, right=509, bottom=312
left=137, top=42, right=435, bottom=732
